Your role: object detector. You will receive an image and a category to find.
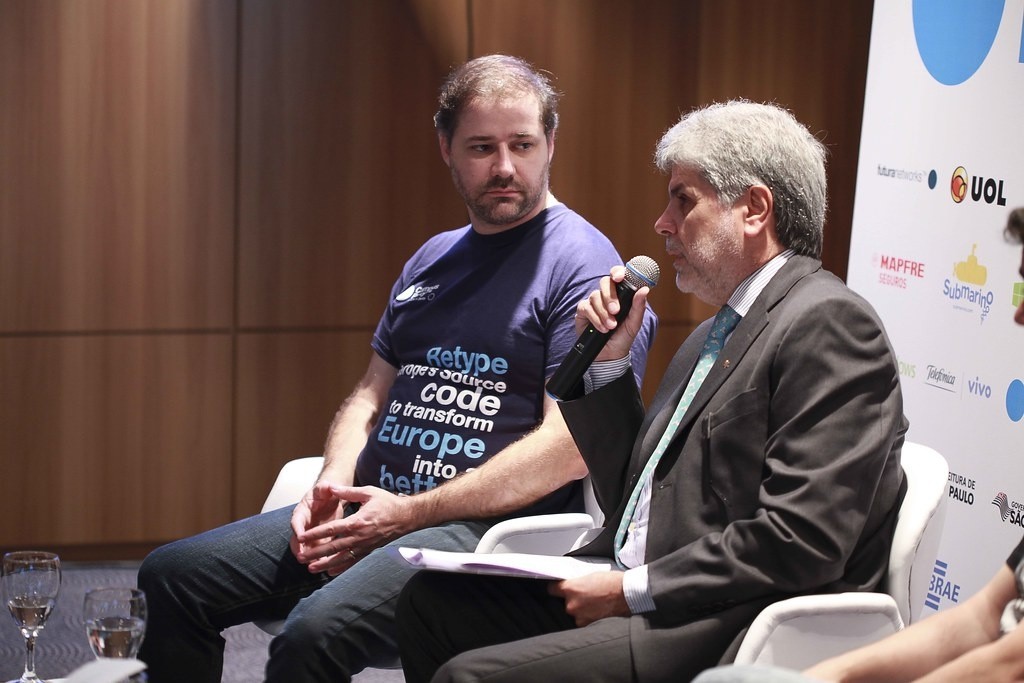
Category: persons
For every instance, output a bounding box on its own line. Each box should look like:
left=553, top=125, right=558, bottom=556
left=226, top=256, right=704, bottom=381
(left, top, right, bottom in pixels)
left=392, top=96, right=915, bottom=682
left=129, top=57, right=658, bottom=683
left=800, top=204, right=1024, bottom=683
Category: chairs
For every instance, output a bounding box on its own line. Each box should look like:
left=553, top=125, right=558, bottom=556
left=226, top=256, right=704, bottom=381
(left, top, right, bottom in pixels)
left=250, top=455, right=605, bottom=670
left=733, top=440, right=950, bottom=674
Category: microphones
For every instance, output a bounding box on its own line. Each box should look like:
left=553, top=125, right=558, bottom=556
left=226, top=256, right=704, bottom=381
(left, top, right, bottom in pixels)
left=545, top=255, right=660, bottom=403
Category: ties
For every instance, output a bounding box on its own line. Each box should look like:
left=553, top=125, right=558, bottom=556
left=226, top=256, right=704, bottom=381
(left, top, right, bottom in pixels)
left=609, top=303, right=744, bottom=572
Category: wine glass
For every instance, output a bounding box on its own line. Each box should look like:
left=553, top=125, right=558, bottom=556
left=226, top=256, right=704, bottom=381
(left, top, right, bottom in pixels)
left=2, top=550, right=62, bottom=683
left=82, top=586, right=148, bottom=661
